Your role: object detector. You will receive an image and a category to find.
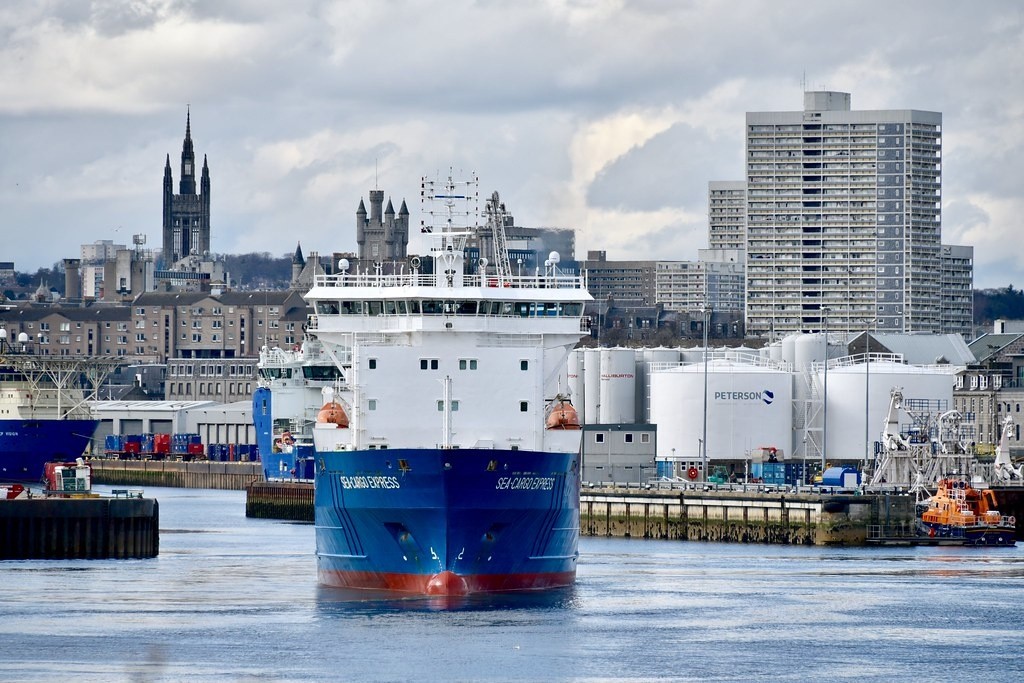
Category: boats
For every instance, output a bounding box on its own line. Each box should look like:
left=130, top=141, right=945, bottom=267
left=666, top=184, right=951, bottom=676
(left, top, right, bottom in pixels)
left=317, top=401, right=349, bottom=429
left=545, top=402, right=580, bottom=431
left=916, top=474, right=1017, bottom=547
left=0, top=355, right=134, bottom=486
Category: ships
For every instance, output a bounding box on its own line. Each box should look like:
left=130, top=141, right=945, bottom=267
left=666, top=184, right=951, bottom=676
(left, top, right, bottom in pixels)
left=302, top=162, right=595, bottom=618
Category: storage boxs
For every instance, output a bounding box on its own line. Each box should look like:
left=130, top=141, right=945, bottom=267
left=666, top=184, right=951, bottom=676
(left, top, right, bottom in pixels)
left=106, top=432, right=259, bottom=461
left=45, top=463, right=93, bottom=498
left=752, top=462, right=813, bottom=487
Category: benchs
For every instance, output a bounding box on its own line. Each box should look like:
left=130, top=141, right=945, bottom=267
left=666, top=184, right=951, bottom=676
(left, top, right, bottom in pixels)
left=112, top=489, right=144, bottom=498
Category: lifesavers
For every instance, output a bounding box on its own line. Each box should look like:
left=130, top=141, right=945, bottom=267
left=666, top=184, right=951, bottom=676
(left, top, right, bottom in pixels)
left=1008, top=516, right=1016, bottom=524
left=689, top=469, right=697, bottom=478
